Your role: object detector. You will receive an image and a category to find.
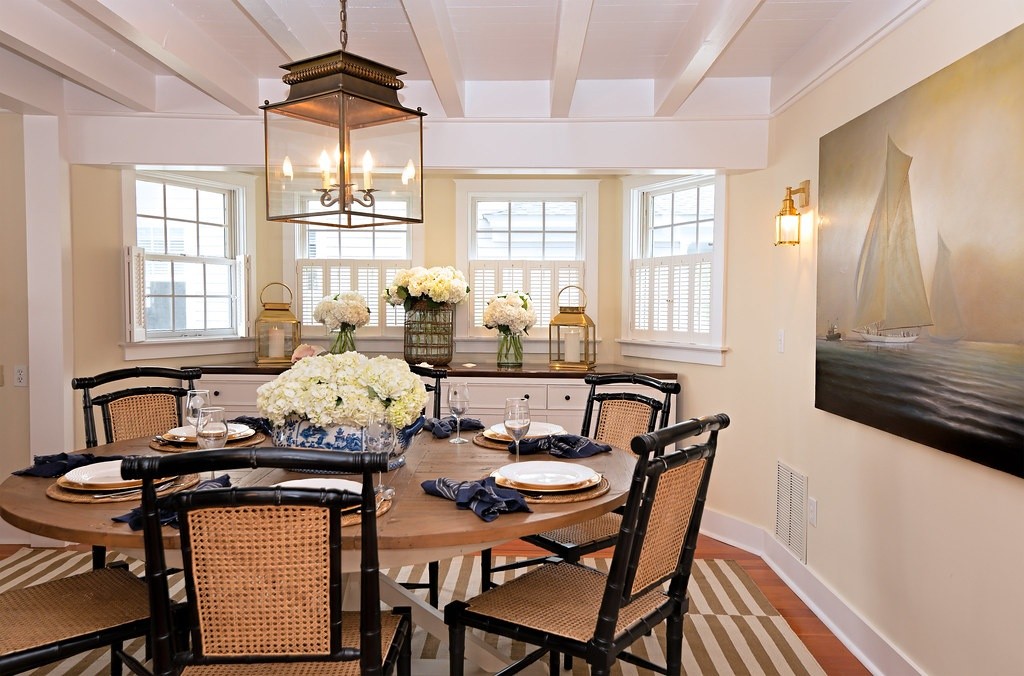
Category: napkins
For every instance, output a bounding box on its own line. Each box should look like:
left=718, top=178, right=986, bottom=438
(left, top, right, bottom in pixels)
left=228, top=415, right=273, bottom=439
left=9, top=452, right=124, bottom=478
left=421, top=476, right=532, bottom=521
left=424, top=416, right=482, bottom=439
left=508, top=434, right=612, bottom=457
left=109, top=473, right=232, bottom=535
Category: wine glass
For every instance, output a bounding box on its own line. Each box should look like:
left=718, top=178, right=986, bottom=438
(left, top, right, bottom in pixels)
left=186, top=390, right=212, bottom=452
left=447, top=381, right=470, bottom=444
left=503, top=398, right=531, bottom=463
left=195, top=407, right=229, bottom=480
left=364, top=409, right=397, bottom=492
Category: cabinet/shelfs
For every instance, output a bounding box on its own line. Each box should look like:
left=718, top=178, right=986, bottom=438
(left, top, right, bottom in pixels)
left=180, top=374, right=676, bottom=507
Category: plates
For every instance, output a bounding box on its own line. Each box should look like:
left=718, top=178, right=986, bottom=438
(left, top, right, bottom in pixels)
left=56, top=460, right=178, bottom=490
left=162, top=423, right=256, bottom=442
left=483, top=422, right=567, bottom=442
left=490, top=460, right=601, bottom=492
left=269, top=478, right=363, bottom=512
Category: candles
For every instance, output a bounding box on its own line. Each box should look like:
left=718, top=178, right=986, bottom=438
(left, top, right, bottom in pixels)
left=267, top=327, right=286, bottom=358
left=565, top=331, right=580, bottom=363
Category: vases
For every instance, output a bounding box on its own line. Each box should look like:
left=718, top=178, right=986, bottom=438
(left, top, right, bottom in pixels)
left=495, top=333, right=523, bottom=367
left=328, top=328, right=358, bottom=353
left=273, top=413, right=425, bottom=469
left=401, top=298, right=456, bottom=365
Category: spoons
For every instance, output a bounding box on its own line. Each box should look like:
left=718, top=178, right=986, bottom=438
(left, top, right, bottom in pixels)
left=111, top=481, right=174, bottom=498
left=152, top=438, right=168, bottom=446
left=376, top=488, right=395, bottom=510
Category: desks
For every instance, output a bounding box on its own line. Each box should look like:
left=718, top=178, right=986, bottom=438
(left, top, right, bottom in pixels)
left=0, top=422, right=637, bottom=676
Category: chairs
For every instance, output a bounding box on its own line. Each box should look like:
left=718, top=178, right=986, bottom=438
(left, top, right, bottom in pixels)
left=71, top=364, right=203, bottom=578
left=121, top=446, right=414, bottom=676
left=444, top=411, right=731, bottom=676
left=0, top=560, right=190, bottom=676
left=483, top=371, right=682, bottom=596
left=398, top=365, right=449, bottom=607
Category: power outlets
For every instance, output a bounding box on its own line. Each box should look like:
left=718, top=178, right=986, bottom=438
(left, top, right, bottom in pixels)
left=13, top=364, right=27, bottom=388
left=808, top=497, right=816, bottom=528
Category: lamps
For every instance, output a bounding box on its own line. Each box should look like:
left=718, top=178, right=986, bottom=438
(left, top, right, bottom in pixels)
left=258, top=0, right=424, bottom=230
left=775, top=179, right=810, bottom=247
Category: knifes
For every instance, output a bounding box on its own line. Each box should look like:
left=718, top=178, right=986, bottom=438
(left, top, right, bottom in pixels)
left=156, top=435, right=182, bottom=448
left=93, top=483, right=185, bottom=498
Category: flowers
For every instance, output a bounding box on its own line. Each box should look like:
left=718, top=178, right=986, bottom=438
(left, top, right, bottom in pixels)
left=255, top=349, right=429, bottom=432
left=482, top=290, right=538, bottom=336
left=315, top=291, right=372, bottom=332
left=379, top=265, right=472, bottom=309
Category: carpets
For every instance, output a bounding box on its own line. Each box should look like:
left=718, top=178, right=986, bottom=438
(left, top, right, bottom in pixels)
left=0, top=547, right=828, bottom=676
